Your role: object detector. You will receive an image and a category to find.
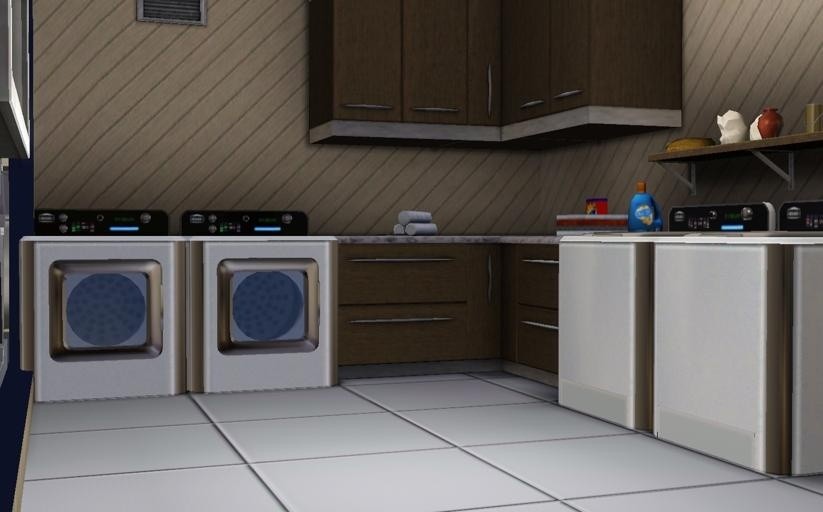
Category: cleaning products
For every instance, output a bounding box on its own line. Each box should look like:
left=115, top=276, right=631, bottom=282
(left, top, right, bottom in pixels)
left=628, top=182, right=663, bottom=232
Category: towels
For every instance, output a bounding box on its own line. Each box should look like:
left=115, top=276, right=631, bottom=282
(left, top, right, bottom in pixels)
left=392, top=224, right=404, bottom=235
left=393, top=224, right=405, bottom=234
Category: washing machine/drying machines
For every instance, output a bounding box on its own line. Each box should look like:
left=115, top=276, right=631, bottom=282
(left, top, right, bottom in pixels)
left=792, top=235, right=823, bottom=477
left=180, top=208, right=339, bottom=394
left=557, top=202, right=776, bottom=431
left=651, top=198, right=823, bottom=477
left=18, top=207, right=188, bottom=403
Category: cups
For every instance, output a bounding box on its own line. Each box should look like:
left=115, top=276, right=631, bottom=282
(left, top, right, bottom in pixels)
left=801, top=104, right=823, bottom=134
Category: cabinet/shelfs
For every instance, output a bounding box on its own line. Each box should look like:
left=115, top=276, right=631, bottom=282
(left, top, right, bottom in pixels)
left=498, top=243, right=560, bottom=386
left=337, top=241, right=504, bottom=378
left=310, top=0, right=500, bottom=144
left=501, top=1, right=683, bottom=140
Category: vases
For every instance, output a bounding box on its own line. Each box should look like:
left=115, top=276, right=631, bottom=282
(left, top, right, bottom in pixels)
left=757, top=108, right=783, bottom=138
